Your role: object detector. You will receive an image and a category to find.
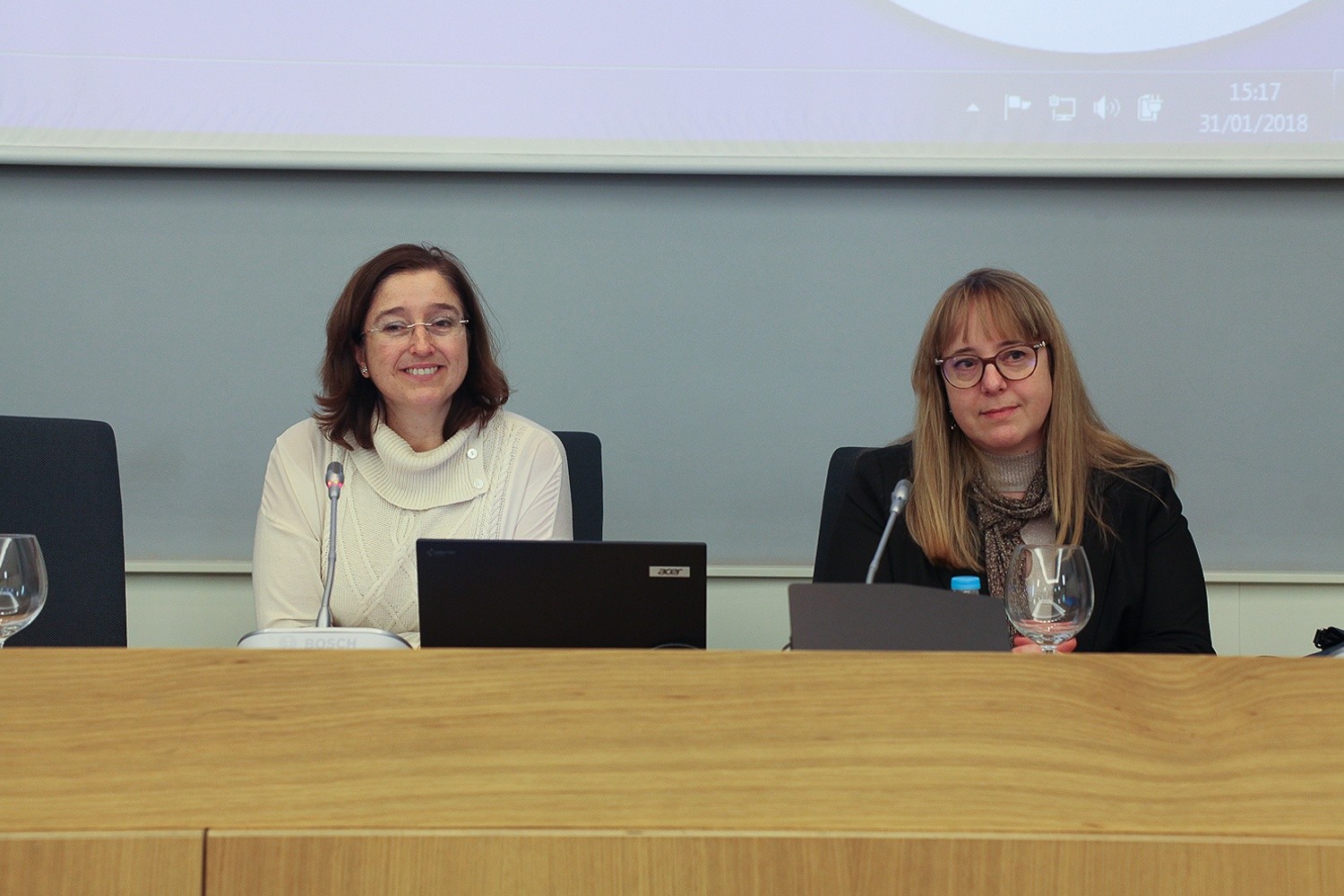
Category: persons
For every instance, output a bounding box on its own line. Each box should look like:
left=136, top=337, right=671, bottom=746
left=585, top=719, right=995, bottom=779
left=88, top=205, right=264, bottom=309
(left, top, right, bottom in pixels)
left=254, top=245, right=573, bottom=650
left=815, top=269, right=1215, bottom=655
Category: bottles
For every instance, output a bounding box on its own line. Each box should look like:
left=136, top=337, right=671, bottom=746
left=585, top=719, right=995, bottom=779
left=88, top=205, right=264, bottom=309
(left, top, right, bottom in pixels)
left=950, top=577, right=981, bottom=596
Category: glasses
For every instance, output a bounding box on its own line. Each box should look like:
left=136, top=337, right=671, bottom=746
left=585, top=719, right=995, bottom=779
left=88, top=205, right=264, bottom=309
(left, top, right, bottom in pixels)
left=934, top=341, right=1049, bottom=389
left=362, top=313, right=471, bottom=335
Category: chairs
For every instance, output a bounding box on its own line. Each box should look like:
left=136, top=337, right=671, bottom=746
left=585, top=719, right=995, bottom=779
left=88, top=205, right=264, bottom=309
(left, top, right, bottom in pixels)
left=552, top=430, right=605, bottom=544
left=0, top=414, right=130, bottom=649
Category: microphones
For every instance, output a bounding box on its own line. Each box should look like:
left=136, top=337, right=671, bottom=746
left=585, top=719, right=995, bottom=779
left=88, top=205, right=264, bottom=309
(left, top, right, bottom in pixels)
left=866, top=479, right=913, bottom=585
left=236, top=462, right=414, bottom=648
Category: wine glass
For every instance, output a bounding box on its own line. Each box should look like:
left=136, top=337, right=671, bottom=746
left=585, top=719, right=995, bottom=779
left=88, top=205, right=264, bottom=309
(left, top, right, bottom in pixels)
left=1004, top=544, right=1094, bottom=654
left=0, top=534, right=48, bottom=649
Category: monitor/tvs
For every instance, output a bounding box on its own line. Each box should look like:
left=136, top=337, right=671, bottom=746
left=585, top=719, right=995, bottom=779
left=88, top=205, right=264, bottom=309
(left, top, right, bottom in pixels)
left=416, top=538, right=707, bottom=651
